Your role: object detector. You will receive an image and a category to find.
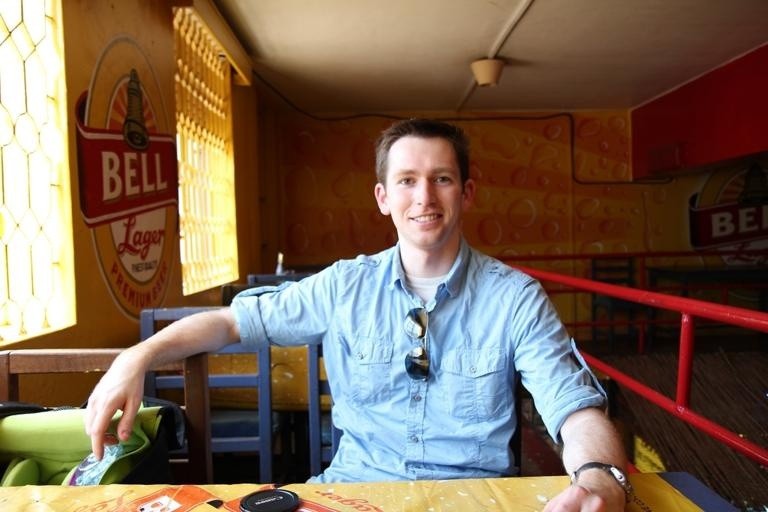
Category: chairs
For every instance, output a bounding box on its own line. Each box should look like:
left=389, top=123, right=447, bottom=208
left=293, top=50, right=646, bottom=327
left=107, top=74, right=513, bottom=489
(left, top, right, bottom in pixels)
left=306, top=343, right=341, bottom=479
left=139, top=303, right=275, bottom=482
left=590, top=256, right=655, bottom=355
left=247, top=272, right=306, bottom=287
left=219, top=283, right=256, bottom=308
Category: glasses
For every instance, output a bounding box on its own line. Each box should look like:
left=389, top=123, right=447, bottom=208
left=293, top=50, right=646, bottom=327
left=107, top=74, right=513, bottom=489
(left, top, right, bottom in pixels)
left=404, top=308, right=431, bottom=382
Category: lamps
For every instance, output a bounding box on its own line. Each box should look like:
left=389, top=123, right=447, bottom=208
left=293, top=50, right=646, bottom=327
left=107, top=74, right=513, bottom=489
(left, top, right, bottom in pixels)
left=471, top=58, right=505, bottom=88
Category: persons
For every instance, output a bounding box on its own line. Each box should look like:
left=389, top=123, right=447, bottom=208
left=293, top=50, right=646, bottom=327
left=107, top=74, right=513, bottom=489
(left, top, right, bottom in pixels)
left=87, top=119, right=628, bottom=512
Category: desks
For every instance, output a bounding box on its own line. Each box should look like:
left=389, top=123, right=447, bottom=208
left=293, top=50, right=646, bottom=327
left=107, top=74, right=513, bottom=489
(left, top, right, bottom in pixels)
left=114, top=338, right=338, bottom=415
left=1, top=471, right=743, bottom=512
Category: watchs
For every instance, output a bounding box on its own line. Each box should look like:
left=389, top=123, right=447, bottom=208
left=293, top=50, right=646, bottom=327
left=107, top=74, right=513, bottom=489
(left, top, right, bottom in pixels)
left=573, top=463, right=630, bottom=497
